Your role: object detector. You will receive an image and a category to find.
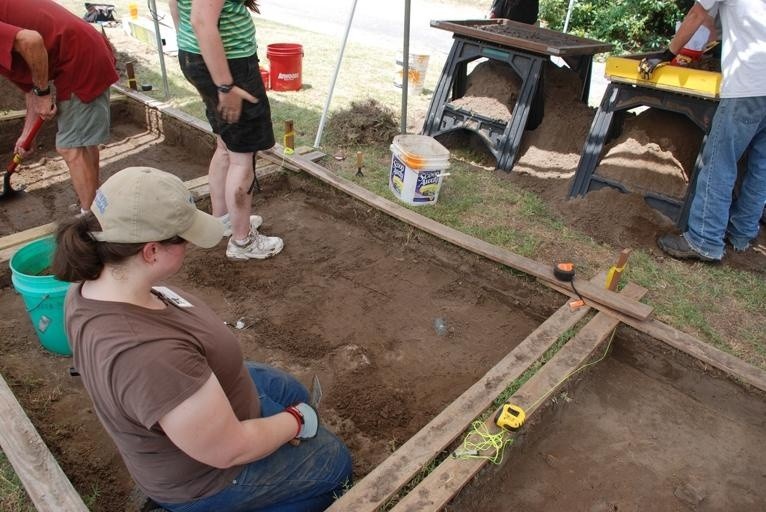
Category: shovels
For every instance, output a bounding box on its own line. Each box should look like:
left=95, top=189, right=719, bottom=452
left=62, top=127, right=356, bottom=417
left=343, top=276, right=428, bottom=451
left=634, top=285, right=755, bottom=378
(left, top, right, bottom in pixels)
left=0, top=82, right=57, bottom=199
left=356, top=151, right=364, bottom=178
left=289, top=373, right=323, bottom=447
left=224, top=317, right=260, bottom=331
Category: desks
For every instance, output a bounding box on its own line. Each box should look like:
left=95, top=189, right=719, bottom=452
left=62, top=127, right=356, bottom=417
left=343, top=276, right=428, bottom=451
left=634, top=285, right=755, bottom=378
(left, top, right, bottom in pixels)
left=421, top=17, right=617, bottom=175
left=567, top=54, right=749, bottom=235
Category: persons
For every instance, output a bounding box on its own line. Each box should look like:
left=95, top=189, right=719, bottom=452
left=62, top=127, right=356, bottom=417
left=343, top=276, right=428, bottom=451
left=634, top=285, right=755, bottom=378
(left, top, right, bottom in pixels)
left=0, top=0, right=120, bottom=218
left=489, top=0, right=540, bottom=25
left=637, top=1, right=766, bottom=263
left=168, top=1, right=285, bottom=262
left=51, top=163, right=354, bottom=511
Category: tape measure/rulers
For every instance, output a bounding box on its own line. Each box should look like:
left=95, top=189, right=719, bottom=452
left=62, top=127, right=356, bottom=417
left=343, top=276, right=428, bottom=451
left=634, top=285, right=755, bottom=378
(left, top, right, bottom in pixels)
left=494, top=402, right=527, bottom=433
left=554, top=262, right=576, bottom=281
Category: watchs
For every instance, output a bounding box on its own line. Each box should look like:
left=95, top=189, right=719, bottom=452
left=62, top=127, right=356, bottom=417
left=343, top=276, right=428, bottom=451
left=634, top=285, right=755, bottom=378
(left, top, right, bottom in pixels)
left=32, top=85, right=50, bottom=96
left=215, top=82, right=235, bottom=93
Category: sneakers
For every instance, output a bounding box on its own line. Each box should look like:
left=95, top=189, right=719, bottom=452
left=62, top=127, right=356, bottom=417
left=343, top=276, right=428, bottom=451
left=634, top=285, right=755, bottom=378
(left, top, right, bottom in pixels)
left=657, top=230, right=724, bottom=262
left=225, top=233, right=285, bottom=262
left=210, top=209, right=263, bottom=237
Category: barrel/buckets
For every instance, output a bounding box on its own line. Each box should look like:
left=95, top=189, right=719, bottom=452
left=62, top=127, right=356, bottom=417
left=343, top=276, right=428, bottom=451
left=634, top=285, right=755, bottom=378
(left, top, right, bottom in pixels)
left=266, top=43, right=304, bottom=92
left=8, top=234, right=79, bottom=356
left=670, top=48, right=705, bottom=66
left=392, top=49, right=431, bottom=96
left=389, top=134, right=451, bottom=207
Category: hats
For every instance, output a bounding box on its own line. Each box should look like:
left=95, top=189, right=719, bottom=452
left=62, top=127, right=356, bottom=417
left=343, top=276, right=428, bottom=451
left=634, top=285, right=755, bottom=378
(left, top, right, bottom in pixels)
left=86, top=166, right=226, bottom=249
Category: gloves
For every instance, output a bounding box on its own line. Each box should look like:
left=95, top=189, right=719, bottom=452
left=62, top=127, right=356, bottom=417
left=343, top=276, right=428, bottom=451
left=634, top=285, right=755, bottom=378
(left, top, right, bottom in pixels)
left=285, top=399, right=321, bottom=440
left=637, top=47, right=676, bottom=79
left=701, top=38, right=724, bottom=58
